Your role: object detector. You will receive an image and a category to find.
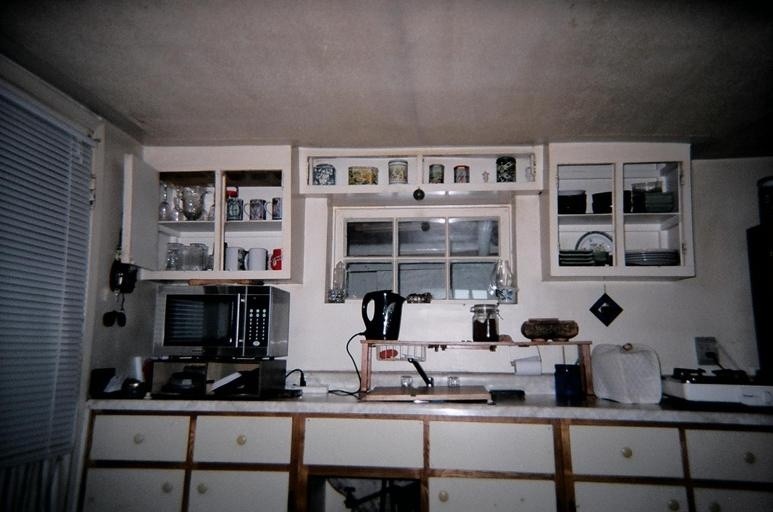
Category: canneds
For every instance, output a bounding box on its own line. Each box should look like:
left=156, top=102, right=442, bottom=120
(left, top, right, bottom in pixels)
left=454, top=166, right=470, bottom=182
left=472, top=304, right=499, bottom=342
left=389, top=159, right=408, bottom=184
left=496, top=157, right=516, bottom=182
left=428, top=164, right=444, bottom=183
left=349, top=167, right=378, bottom=184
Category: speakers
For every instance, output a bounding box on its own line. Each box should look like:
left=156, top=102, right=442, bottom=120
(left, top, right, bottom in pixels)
left=555, top=364, right=586, bottom=408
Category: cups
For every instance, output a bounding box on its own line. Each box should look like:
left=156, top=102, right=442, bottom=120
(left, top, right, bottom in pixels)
left=224, top=247, right=242, bottom=271
left=264, top=197, right=282, bottom=220
left=243, top=199, right=267, bottom=220
left=244, top=247, right=268, bottom=271
left=270, top=249, right=281, bottom=270
left=400, top=376, right=411, bottom=394
left=165, top=241, right=209, bottom=271
left=227, top=199, right=243, bottom=221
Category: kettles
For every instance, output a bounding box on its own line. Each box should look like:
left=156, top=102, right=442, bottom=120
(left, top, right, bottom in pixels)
left=362, top=289, right=406, bottom=340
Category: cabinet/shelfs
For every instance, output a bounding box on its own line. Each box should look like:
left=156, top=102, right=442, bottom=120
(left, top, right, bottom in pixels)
left=298, top=414, right=561, bottom=512
left=541, top=141, right=696, bottom=281
left=297, top=145, right=544, bottom=193
left=119, top=143, right=297, bottom=282
left=77, top=409, right=298, bottom=512
left=560, top=418, right=773, bottom=512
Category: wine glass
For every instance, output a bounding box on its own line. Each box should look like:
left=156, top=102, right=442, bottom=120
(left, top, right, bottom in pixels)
left=160, top=181, right=215, bottom=223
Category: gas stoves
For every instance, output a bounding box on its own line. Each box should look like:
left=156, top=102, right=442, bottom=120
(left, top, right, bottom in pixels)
left=660, top=366, right=773, bottom=385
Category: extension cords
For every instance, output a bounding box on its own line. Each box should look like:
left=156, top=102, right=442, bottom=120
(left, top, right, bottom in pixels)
left=284, top=383, right=328, bottom=395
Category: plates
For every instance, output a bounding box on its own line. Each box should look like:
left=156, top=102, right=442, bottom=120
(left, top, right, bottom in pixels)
left=558, top=230, right=614, bottom=267
left=623, top=181, right=678, bottom=214
left=626, top=249, right=681, bottom=266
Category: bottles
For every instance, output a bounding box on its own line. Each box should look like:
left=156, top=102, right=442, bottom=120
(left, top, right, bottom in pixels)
left=446, top=377, right=459, bottom=388
left=554, top=365, right=583, bottom=407
left=469, top=303, right=503, bottom=342
left=487, top=260, right=513, bottom=297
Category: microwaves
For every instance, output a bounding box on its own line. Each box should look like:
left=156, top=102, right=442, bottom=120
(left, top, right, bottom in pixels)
left=152, top=284, right=289, bottom=360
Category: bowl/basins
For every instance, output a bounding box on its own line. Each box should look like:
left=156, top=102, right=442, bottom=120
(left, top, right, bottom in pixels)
left=557, top=188, right=613, bottom=214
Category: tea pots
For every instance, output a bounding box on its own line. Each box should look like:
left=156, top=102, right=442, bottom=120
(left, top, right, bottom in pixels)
left=174, top=185, right=209, bottom=221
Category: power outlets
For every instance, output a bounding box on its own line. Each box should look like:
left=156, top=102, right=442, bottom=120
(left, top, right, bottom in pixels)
left=695, top=337, right=718, bottom=365
